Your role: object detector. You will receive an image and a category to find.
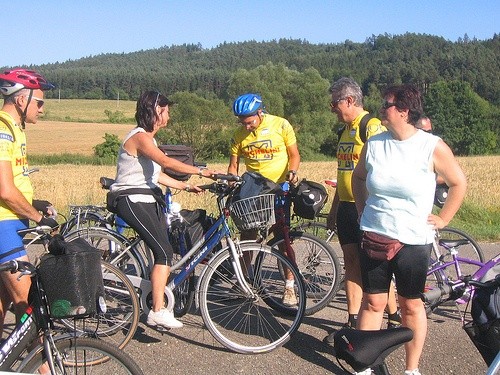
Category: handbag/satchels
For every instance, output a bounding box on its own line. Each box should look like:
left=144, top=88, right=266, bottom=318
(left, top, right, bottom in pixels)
left=233, top=172, right=283, bottom=223
left=159, top=142, right=193, bottom=180
left=360, top=231, right=404, bottom=261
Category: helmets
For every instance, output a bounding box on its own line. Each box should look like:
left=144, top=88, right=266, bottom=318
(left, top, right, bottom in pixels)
left=233, top=94, right=262, bottom=116
left=0, top=68, right=55, bottom=95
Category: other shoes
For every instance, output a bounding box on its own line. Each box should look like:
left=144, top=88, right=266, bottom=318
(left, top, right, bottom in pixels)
left=404, top=369, right=421, bottom=375
left=354, top=368, right=373, bottom=375
left=388, top=312, right=403, bottom=329
left=325, top=319, right=355, bottom=343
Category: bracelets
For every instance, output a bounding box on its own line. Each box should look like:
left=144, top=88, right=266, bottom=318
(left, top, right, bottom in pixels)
left=186, top=185, right=190, bottom=190
left=199, top=168, right=203, bottom=176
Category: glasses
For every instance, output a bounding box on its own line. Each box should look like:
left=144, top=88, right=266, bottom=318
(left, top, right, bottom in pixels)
left=237, top=115, right=258, bottom=126
left=382, top=101, right=395, bottom=109
left=419, top=128, right=433, bottom=134
left=154, top=88, right=162, bottom=110
left=19, top=94, right=44, bottom=109
left=330, top=95, right=354, bottom=109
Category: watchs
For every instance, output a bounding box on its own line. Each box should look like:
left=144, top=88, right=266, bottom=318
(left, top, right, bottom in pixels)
left=289, top=170, right=296, bottom=175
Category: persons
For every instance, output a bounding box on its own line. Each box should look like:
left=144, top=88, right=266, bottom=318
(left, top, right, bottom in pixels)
left=322, top=77, right=402, bottom=347
left=415, top=116, right=445, bottom=184
left=0, top=68, right=56, bottom=375
left=351, top=85, right=468, bottom=375
left=109, top=91, right=225, bottom=329
left=227, top=94, right=300, bottom=305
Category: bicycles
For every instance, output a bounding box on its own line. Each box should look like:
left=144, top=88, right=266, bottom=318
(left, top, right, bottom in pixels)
left=276, top=177, right=486, bottom=297
left=334, top=275, right=500, bottom=375
left=0, top=175, right=342, bottom=375
left=421, top=240, right=500, bottom=328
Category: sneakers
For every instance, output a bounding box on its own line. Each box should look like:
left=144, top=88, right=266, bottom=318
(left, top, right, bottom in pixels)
left=146, top=308, right=183, bottom=328
left=228, top=281, right=245, bottom=299
left=283, top=285, right=297, bottom=306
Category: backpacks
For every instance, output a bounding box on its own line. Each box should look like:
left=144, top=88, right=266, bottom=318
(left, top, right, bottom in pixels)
left=169, top=206, right=222, bottom=258
left=471, top=274, right=500, bottom=363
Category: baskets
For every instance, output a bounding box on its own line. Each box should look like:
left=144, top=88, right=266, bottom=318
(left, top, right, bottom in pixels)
left=36, top=251, right=106, bottom=319
left=462, top=318, right=500, bottom=367
left=230, top=194, right=276, bottom=232
